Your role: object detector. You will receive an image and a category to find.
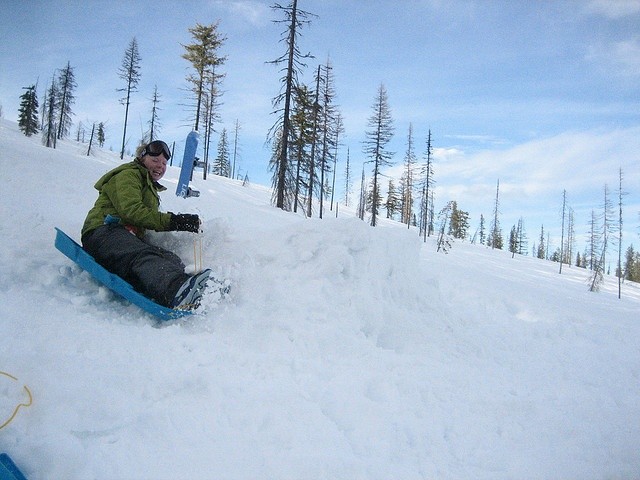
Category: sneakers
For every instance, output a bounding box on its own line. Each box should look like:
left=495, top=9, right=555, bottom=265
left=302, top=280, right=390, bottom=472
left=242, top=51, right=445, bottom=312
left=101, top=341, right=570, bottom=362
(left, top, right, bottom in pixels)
left=170, top=267, right=214, bottom=311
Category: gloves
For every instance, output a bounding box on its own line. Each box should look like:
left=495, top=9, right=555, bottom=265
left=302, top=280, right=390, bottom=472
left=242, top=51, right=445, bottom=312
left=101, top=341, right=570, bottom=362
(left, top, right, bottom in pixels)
left=171, top=212, right=203, bottom=233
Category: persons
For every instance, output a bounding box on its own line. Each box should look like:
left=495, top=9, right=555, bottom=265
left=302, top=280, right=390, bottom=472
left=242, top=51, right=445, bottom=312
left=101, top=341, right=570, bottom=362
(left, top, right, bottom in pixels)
left=81, top=140, right=232, bottom=311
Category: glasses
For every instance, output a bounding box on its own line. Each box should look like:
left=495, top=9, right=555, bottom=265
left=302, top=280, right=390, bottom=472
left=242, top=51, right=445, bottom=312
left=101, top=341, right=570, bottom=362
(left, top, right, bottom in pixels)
left=140, top=140, right=171, bottom=160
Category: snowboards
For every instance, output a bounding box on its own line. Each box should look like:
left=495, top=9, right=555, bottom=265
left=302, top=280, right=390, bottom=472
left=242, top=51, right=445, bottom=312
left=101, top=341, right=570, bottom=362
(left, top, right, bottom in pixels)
left=175, top=131, right=205, bottom=198
